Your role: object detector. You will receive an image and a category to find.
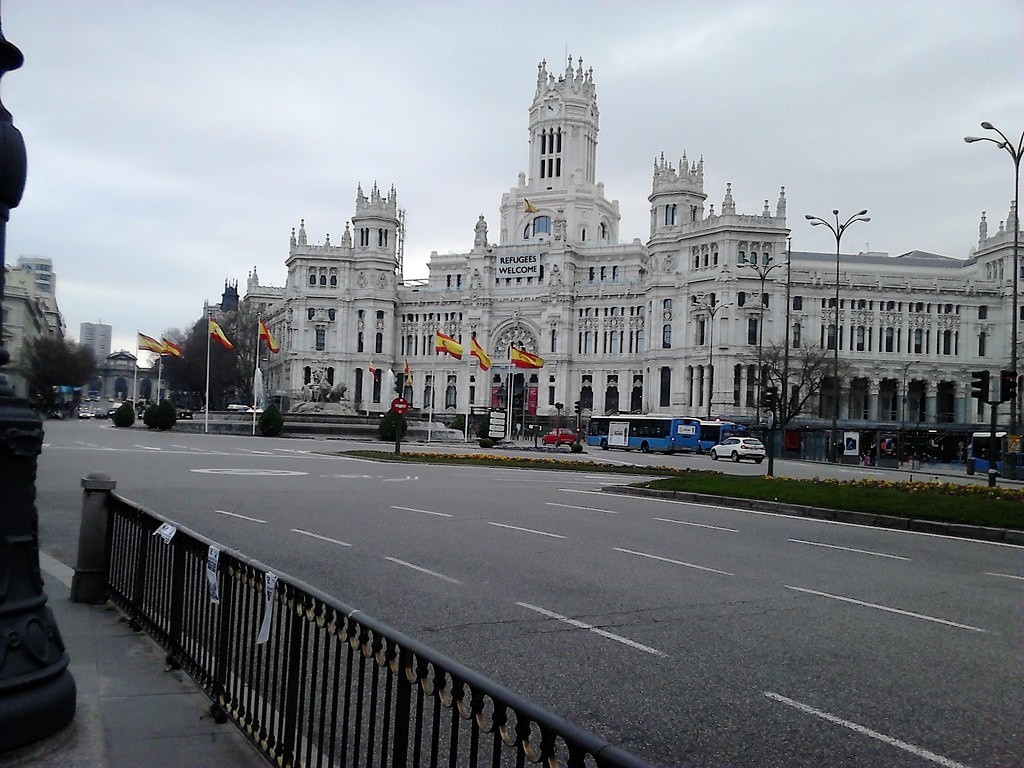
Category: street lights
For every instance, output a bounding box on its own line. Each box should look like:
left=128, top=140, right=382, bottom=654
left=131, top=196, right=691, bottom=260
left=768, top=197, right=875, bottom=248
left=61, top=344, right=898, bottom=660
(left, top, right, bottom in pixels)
left=901, top=360, right=921, bottom=465
left=690, top=299, right=734, bottom=421
left=963, top=122, right=1024, bottom=435
left=805, top=209, right=872, bottom=464
left=737, top=256, right=789, bottom=436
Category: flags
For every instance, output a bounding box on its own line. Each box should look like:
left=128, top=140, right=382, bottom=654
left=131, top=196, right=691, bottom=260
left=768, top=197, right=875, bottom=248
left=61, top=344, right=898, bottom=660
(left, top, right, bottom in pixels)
left=524, top=198, right=539, bottom=214
left=434, top=329, right=463, bottom=360
left=369, top=359, right=379, bottom=382
left=208, top=316, right=234, bottom=349
left=469, top=334, right=493, bottom=372
left=404, top=357, right=413, bottom=385
left=161, top=338, right=185, bottom=360
left=510, top=346, right=545, bottom=369
left=258, top=317, right=279, bottom=353
left=138, top=333, right=164, bottom=355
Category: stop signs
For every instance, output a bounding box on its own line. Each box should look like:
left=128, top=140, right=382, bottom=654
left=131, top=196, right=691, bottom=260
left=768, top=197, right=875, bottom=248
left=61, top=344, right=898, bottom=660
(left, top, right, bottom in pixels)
left=392, top=398, right=409, bottom=414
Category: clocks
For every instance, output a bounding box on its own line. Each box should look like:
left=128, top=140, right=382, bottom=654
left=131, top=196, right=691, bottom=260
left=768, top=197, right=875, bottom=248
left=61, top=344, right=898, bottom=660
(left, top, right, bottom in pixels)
left=545, top=103, right=560, bottom=117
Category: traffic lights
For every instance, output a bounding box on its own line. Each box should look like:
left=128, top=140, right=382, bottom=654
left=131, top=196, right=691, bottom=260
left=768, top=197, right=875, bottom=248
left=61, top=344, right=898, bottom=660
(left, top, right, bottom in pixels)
left=574, top=401, right=581, bottom=413
left=394, top=372, right=404, bottom=393
left=999, top=369, right=1018, bottom=401
left=971, top=370, right=990, bottom=401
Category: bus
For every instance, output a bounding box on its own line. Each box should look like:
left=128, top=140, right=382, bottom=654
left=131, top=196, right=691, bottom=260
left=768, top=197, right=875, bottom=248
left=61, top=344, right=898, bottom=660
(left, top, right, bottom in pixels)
left=587, top=413, right=701, bottom=454
left=696, top=420, right=746, bottom=455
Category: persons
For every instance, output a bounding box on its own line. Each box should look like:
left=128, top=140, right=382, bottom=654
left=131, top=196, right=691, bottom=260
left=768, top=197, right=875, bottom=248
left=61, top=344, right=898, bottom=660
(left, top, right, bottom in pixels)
left=867, top=441, right=878, bottom=466
left=463, top=424, right=481, bottom=439
left=835, top=440, right=845, bottom=463
left=513, top=428, right=534, bottom=441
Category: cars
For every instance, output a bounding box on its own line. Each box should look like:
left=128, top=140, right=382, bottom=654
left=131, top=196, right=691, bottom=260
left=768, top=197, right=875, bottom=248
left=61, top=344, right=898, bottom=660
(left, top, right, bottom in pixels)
left=50, top=391, right=151, bottom=419
left=227, top=404, right=264, bottom=412
left=176, top=410, right=194, bottom=420
left=710, top=437, right=766, bottom=464
left=541, top=427, right=575, bottom=447
